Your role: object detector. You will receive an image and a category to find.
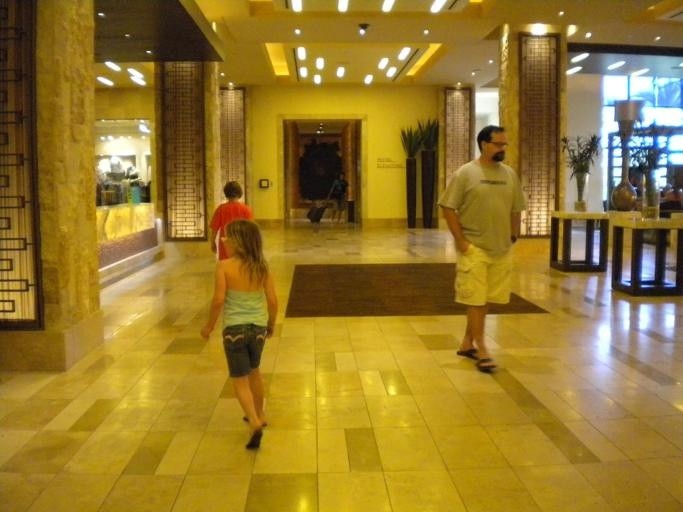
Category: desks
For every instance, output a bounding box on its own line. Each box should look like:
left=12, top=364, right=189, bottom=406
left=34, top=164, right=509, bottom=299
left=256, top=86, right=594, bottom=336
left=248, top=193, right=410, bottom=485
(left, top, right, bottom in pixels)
left=611, top=215, right=683, bottom=298
left=549, top=210, right=610, bottom=270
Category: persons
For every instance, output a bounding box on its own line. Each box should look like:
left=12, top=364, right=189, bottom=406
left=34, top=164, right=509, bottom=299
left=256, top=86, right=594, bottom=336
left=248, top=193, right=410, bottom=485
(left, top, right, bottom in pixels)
left=201, top=220, right=278, bottom=448
left=209, top=183, right=256, bottom=261
left=327, top=172, right=349, bottom=223
left=437, top=124, right=528, bottom=369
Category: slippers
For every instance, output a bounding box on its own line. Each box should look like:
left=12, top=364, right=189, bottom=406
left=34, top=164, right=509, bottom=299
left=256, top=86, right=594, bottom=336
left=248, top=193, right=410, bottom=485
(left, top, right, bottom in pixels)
left=457, top=348, right=479, bottom=359
left=476, top=358, right=496, bottom=370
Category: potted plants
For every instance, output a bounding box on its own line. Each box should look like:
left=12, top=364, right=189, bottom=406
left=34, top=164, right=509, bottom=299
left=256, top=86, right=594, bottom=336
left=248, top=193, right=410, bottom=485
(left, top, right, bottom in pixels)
left=626, top=121, right=676, bottom=220
left=558, top=130, right=602, bottom=209
left=386, top=118, right=441, bottom=228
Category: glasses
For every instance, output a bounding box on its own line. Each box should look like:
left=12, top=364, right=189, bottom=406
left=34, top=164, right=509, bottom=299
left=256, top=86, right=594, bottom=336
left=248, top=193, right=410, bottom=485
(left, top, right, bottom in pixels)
left=489, top=141, right=508, bottom=147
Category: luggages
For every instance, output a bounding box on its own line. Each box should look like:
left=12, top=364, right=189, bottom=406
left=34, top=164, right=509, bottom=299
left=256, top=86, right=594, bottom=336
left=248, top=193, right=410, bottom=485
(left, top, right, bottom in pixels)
left=307, top=197, right=329, bottom=223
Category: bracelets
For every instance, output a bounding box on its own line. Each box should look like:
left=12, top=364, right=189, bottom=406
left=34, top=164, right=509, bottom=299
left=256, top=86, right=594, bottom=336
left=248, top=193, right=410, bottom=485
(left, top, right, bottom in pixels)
left=510, top=236, right=517, bottom=243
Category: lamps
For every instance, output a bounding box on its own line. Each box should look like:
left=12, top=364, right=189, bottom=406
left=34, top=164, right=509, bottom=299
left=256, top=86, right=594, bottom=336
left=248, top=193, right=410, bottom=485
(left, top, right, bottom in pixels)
left=614, top=75, right=645, bottom=122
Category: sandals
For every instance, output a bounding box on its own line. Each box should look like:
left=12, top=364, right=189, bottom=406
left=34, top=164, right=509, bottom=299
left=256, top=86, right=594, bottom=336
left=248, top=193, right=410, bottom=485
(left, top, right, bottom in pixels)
left=244, top=413, right=267, bottom=449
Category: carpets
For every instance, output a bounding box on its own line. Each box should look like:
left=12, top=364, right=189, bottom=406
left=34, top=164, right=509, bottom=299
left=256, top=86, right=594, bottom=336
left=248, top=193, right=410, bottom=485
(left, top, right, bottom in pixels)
left=285, top=260, right=553, bottom=318
